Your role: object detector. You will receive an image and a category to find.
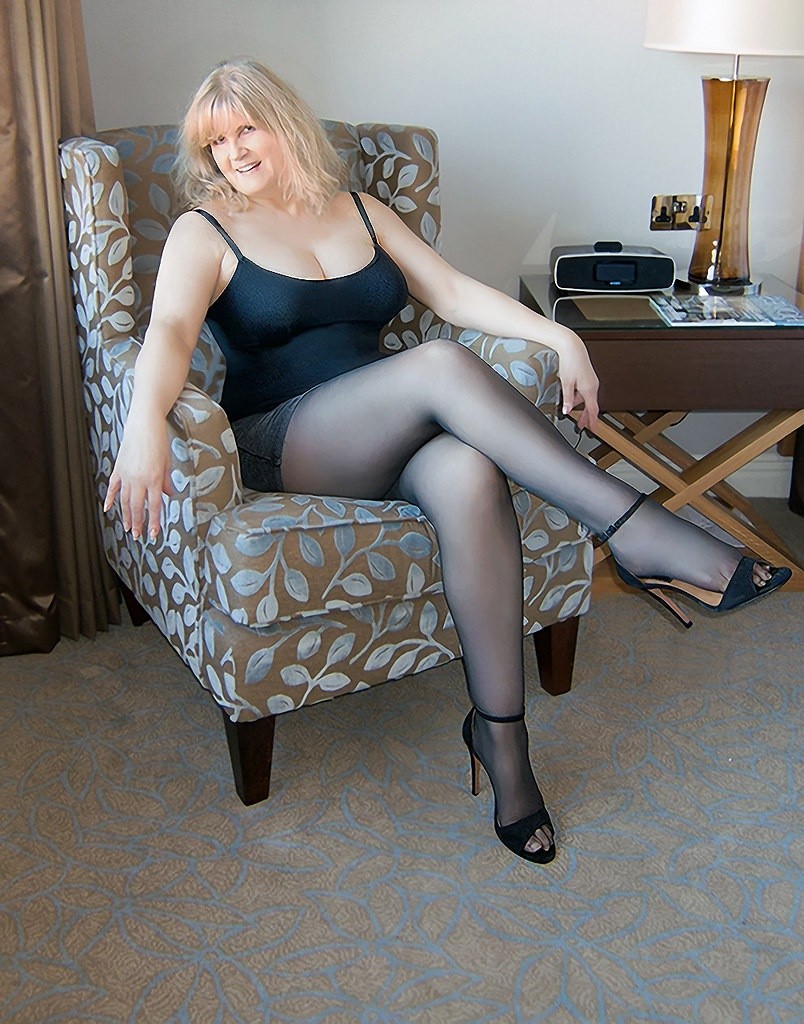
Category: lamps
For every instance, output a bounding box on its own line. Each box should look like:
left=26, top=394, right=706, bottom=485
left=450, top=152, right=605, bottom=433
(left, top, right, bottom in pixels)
left=642, top=0, right=804, bottom=295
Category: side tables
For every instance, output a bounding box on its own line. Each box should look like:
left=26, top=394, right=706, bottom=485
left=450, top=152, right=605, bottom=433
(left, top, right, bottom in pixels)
left=517, top=272, right=804, bottom=594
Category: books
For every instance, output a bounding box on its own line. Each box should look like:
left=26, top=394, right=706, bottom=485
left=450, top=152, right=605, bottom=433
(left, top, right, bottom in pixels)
left=648, top=292, right=804, bottom=327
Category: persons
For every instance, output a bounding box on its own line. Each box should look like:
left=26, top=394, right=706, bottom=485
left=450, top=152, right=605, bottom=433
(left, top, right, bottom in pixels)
left=103, top=60, right=793, bottom=864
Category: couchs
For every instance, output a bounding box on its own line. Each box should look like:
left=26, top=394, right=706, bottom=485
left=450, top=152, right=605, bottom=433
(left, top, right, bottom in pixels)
left=56, top=119, right=591, bottom=804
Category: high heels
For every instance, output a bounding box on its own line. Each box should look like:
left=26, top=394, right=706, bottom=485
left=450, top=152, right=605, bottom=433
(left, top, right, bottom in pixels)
left=462, top=707, right=556, bottom=865
left=598, top=493, right=792, bottom=629
